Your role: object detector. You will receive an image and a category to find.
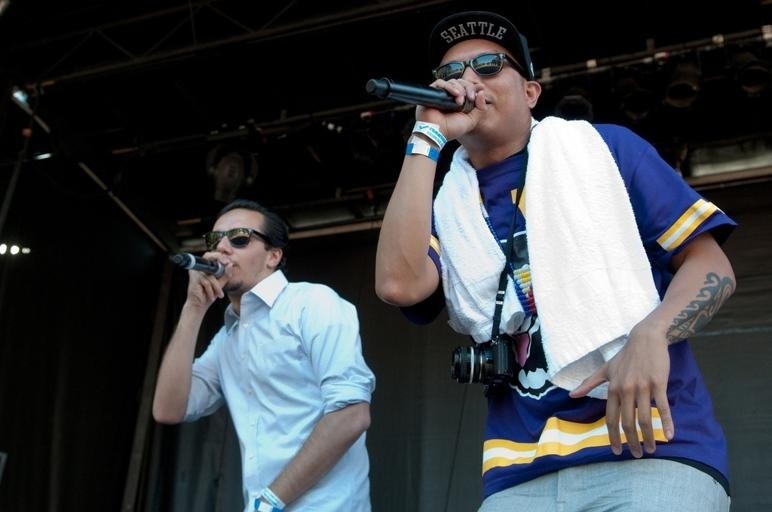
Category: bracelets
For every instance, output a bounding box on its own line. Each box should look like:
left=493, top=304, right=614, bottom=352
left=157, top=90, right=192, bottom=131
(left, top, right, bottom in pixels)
left=405, top=120, right=446, bottom=162
left=261, top=486, right=286, bottom=510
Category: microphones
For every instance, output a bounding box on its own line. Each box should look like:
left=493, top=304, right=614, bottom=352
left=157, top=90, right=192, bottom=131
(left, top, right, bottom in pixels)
left=366, top=75, right=476, bottom=114
left=169, top=252, right=225, bottom=278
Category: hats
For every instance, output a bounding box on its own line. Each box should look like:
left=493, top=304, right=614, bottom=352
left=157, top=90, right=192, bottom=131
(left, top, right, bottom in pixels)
left=425, top=10, right=536, bottom=78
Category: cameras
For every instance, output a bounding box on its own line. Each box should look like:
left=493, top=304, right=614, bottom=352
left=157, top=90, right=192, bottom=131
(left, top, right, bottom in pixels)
left=449, top=332, right=520, bottom=388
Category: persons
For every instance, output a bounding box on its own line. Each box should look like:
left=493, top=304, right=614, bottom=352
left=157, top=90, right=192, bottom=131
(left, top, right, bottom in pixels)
left=372, top=8, right=743, bottom=512
left=150, top=200, right=377, bottom=512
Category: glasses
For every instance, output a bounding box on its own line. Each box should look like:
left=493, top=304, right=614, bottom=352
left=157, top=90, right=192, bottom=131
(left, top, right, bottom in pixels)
left=201, top=226, right=276, bottom=253
left=430, top=51, right=529, bottom=83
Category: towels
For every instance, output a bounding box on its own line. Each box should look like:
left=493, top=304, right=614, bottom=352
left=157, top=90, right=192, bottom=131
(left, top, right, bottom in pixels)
left=430, top=111, right=664, bottom=406
